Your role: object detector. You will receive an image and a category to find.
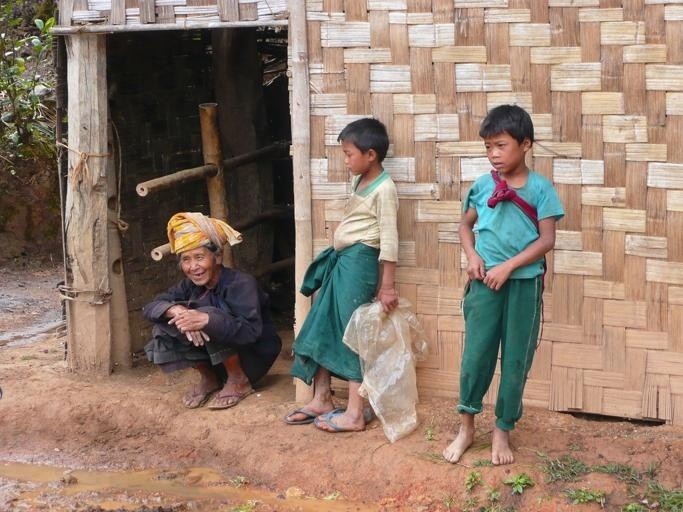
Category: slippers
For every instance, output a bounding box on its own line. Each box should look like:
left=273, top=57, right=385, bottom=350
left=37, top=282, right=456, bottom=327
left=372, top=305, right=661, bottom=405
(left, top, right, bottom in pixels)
left=207, top=387, right=256, bottom=411
left=281, top=402, right=333, bottom=426
left=314, top=407, right=372, bottom=435
left=181, top=382, right=220, bottom=411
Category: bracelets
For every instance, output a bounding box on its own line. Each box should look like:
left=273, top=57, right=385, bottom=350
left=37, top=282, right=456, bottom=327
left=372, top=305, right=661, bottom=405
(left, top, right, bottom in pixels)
left=379, top=286, right=396, bottom=296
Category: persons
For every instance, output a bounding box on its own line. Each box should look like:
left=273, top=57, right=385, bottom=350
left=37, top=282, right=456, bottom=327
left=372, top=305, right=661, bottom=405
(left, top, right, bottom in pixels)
left=140, top=211, right=283, bottom=409
left=277, top=118, right=399, bottom=432
left=442, top=105, right=565, bottom=466
